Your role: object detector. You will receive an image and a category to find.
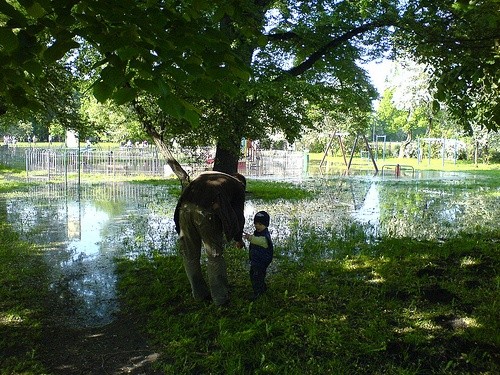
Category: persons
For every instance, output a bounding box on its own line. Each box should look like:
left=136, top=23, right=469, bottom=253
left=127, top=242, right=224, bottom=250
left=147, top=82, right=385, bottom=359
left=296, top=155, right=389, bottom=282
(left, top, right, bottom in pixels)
left=398, top=129, right=413, bottom=159
left=241, top=210, right=274, bottom=294
left=0, top=125, right=307, bottom=175
left=173, top=171, right=247, bottom=306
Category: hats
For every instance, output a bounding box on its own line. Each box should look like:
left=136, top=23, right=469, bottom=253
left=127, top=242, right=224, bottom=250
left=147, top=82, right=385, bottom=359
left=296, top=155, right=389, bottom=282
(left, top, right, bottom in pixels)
left=254, top=211, right=269, bottom=227
